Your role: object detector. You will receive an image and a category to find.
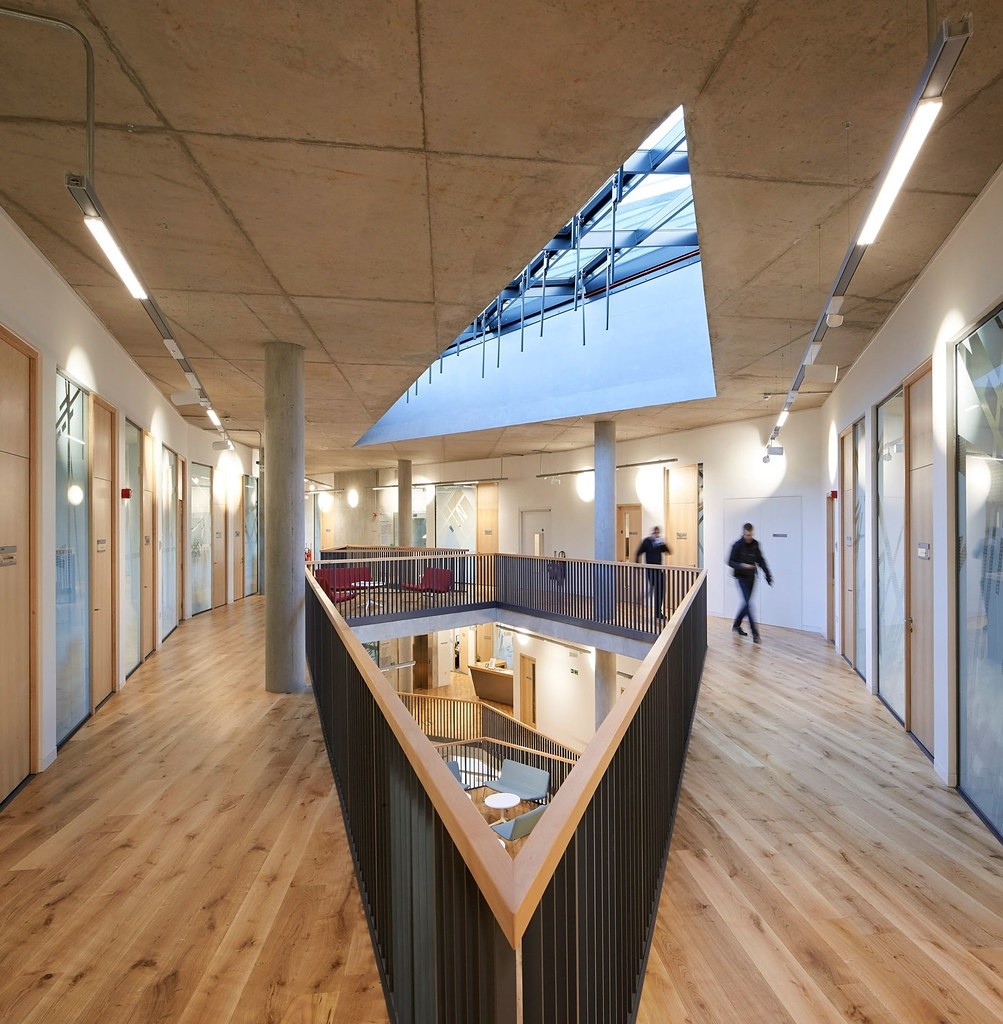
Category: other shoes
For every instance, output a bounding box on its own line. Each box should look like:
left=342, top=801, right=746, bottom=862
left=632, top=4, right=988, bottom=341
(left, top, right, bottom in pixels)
left=733, top=624, right=748, bottom=636
left=656, top=613, right=667, bottom=620
left=753, top=635, right=761, bottom=644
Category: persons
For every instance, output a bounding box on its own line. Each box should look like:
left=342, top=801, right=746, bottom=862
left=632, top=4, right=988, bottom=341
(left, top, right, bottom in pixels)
left=973, top=526, right=1003, bottom=630
left=634, top=526, right=671, bottom=619
left=727, top=522, right=772, bottom=644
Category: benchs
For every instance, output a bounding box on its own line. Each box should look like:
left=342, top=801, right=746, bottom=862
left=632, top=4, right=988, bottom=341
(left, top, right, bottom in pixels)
left=315, top=577, right=356, bottom=617
left=490, top=805, right=547, bottom=841
left=486, top=759, right=550, bottom=801
left=316, top=568, right=373, bottom=591
left=447, top=762, right=470, bottom=790
left=402, top=568, right=452, bottom=602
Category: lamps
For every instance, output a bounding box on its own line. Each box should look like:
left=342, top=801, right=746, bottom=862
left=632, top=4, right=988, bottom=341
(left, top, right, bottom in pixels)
left=768, top=11, right=975, bottom=439
left=65, top=171, right=241, bottom=452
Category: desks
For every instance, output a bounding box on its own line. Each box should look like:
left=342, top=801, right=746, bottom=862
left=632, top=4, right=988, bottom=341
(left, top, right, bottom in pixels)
left=351, top=581, right=386, bottom=611
left=485, top=792, right=521, bottom=827
